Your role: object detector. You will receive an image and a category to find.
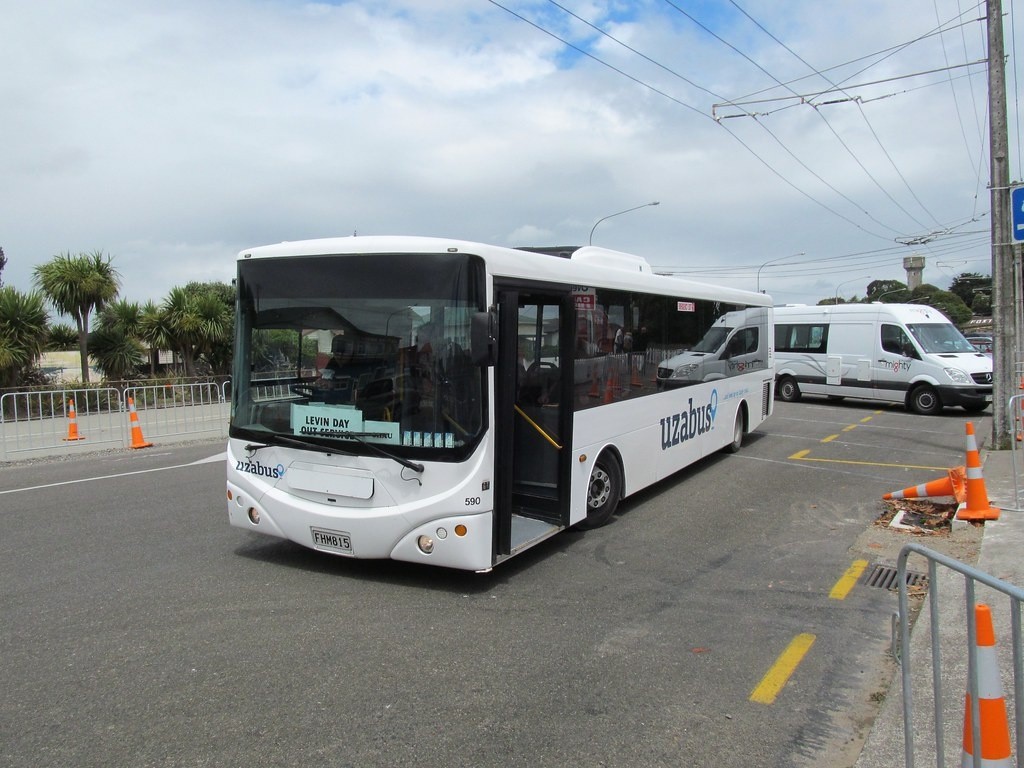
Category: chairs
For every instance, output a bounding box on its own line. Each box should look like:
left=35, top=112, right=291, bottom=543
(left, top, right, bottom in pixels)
left=524, top=362, right=567, bottom=406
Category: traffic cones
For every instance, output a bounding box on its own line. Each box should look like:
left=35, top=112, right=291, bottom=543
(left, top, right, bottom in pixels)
left=62, top=400, right=86, bottom=441
left=601, top=368, right=616, bottom=404
left=959, top=604, right=1015, bottom=768
left=587, top=369, right=601, bottom=396
left=1019, top=375, right=1024, bottom=390
left=1016, top=398, right=1024, bottom=441
left=127, top=397, right=153, bottom=449
left=630, top=357, right=642, bottom=386
left=957, top=420, right=1001, bottom=523
left=882, top=466, right=966, bottom=506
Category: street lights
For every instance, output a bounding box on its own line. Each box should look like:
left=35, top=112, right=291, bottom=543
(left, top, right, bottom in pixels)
left=877, top=287, right=906, bottom=303
left=835, top=275, right=872, bottom=304
left=589, top=200, right=661, bottom=245
left=757, top=251, right=806, bottom=294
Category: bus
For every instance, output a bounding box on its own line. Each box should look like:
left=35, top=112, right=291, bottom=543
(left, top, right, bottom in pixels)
left=225, top=236, right=777, bottom=575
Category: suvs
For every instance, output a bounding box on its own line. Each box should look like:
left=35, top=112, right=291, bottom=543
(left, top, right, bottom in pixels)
left=963, top=336, right=994, bottom=354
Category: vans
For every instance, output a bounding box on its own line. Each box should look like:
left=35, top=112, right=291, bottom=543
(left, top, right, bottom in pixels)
left=775, top=302, right=995, bottom=416
left=654, top=308, right=768, bottom=391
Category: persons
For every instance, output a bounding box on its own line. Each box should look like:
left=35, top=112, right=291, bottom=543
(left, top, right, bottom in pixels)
left=309, top=335, right=379, bottom=409
left=418, top=322, right=478, bottom=448
left=575, top=326, right=651, bottom=404
